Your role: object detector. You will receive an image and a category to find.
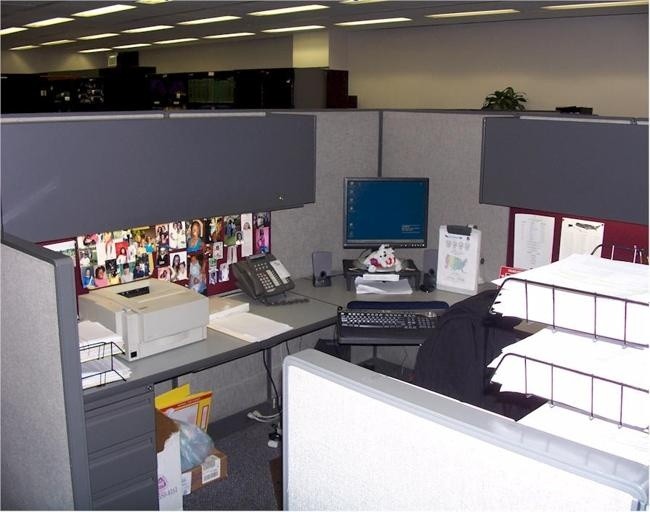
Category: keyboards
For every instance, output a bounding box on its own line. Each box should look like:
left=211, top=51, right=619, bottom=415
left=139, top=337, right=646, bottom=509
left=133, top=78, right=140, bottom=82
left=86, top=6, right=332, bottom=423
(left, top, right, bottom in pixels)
left=336, top=309, right=440, bottom=329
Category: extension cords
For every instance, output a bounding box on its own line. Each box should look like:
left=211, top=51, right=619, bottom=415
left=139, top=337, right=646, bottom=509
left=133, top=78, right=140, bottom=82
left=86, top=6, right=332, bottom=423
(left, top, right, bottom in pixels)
left=268, top=427, right=282, bottom=448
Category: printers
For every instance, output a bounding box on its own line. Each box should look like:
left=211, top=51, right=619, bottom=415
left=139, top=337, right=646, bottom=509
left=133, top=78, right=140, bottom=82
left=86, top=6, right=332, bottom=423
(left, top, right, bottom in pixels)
left=78, top=277, right=210, bottom=362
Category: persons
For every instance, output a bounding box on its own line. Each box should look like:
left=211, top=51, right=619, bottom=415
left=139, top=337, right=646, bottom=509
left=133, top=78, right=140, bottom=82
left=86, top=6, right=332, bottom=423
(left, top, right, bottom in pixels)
left=80, top=213, right=267, bottom=293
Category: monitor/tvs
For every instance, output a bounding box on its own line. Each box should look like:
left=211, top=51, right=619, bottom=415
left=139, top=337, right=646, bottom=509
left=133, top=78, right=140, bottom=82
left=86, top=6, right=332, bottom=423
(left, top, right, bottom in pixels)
left=343, top=176, right=430, bottom=270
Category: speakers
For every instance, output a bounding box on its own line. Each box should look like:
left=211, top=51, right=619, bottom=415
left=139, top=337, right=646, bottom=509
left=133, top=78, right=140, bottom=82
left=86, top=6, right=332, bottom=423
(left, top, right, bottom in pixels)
left=423, top=248, right=438, bottom=276
left=313, top=251, right=332, bottom=287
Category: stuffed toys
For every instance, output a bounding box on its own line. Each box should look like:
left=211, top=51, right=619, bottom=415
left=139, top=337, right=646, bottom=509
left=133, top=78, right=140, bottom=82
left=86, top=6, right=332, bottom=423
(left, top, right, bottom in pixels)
left=361, top=243, right=402, bottom=273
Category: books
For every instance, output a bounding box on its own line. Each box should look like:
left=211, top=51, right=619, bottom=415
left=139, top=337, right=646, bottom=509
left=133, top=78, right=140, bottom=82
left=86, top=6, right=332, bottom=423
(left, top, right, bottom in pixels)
left=207, top=312, right=294, bottom=343
left=208, top=295, right=250, bottom=320
left=436, top=225, right=482, bottom=291
left=77, top=320, right=133, bottom=391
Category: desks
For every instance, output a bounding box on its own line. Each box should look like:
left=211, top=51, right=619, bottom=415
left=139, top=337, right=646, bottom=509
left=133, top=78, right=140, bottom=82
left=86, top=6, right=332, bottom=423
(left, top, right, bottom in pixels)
left=83, top=291, right=344, bottom=510
left=287, top=276, right=546, bottom=342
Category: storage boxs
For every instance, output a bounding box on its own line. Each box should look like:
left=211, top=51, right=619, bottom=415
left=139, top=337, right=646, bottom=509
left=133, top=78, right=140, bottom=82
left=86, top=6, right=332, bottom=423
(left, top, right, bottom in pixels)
left=181, top=448, right=230, bottom=498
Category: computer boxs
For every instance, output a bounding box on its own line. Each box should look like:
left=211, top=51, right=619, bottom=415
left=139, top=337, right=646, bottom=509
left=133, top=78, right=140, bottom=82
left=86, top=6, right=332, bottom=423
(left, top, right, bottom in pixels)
left=315, top=339, right=352, bottom=362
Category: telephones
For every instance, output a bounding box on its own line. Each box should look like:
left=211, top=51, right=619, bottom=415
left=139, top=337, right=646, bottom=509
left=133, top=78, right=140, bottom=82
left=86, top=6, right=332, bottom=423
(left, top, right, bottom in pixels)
left=231, top=252, right=294, bottom=300
